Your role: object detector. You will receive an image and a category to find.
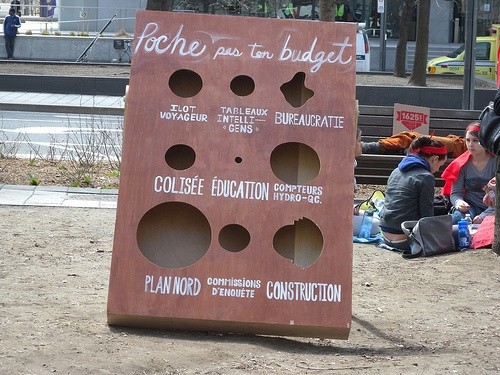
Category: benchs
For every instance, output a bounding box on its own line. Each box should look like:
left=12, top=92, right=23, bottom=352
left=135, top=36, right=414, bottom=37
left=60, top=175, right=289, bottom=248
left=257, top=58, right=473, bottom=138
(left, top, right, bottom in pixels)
left=354, top=104, right=482, bottom=187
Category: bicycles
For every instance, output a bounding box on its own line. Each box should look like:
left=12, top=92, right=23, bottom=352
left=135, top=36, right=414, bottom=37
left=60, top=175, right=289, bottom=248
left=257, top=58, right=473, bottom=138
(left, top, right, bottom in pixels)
left=111, top=39, right=133, bottom=63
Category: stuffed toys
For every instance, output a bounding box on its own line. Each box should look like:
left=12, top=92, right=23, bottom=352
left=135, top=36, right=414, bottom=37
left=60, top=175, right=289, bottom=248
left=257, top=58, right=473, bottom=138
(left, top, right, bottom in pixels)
left=472, top=177, right=496, bottom=225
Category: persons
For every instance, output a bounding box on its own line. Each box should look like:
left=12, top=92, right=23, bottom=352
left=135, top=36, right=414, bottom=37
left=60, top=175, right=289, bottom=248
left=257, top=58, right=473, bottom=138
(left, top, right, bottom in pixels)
left=449, top=122, right=498, bottom=227
left=354, top=4, right=362, bottom=22
left=377, top=136, right=480, bottom=252
left=3, top=8, right=21, bottom=60
left=11, top=0, right=20, bottom=16
left=360, top=130, right=467, bottom=158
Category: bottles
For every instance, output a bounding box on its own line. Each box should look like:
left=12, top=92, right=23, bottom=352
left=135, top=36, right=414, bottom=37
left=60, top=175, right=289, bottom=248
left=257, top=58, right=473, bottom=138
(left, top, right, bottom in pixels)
left=464, top=214, right=472, bottom=235
left=458, top=221, right=471, bottom=252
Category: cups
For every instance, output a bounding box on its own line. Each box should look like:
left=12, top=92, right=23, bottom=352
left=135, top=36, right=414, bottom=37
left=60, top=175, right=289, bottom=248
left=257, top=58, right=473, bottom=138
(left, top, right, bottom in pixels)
left=359, top=210, right=376, bottom=239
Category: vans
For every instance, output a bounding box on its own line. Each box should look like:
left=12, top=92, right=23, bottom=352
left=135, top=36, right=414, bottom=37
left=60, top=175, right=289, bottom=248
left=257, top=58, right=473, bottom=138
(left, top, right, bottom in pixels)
left=356, top=22, right=372, bottom=72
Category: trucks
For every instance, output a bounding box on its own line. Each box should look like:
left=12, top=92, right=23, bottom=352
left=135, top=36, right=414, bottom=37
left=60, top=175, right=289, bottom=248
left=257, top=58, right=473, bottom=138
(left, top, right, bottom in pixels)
left=426, top=24, right=500, bottom=79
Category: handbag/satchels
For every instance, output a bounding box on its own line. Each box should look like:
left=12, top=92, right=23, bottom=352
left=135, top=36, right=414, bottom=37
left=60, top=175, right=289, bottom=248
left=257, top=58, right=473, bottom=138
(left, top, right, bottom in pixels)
left=401, top=214, right=456, bottom=259
left=478, top=101, right=500, bottom=156
